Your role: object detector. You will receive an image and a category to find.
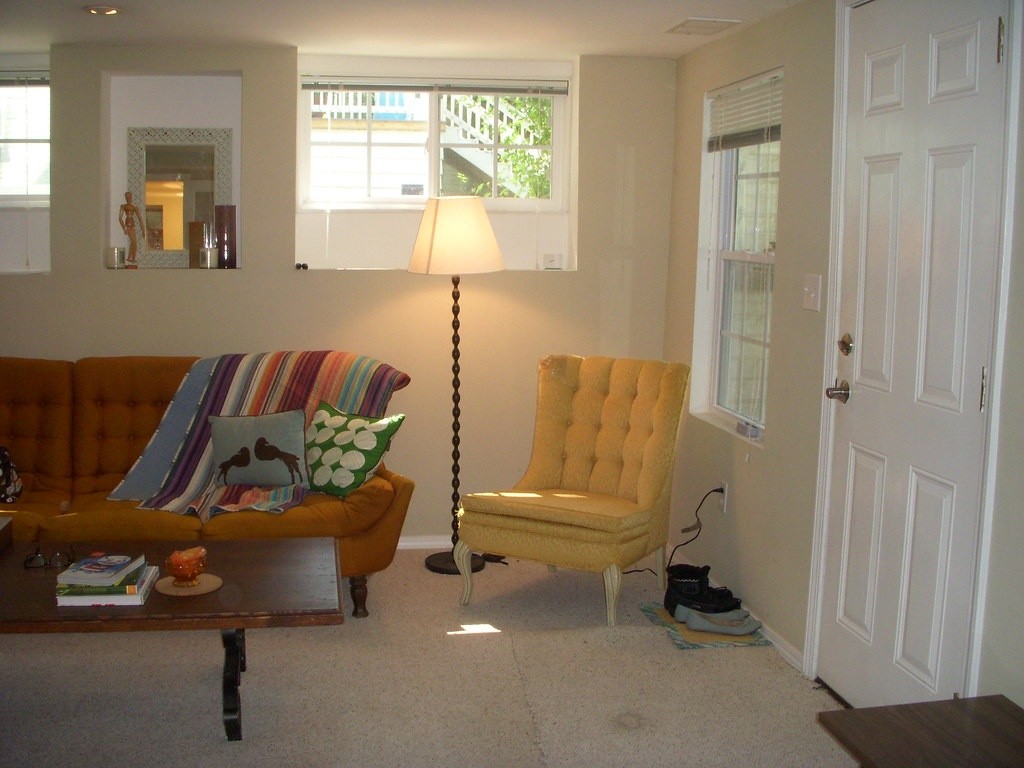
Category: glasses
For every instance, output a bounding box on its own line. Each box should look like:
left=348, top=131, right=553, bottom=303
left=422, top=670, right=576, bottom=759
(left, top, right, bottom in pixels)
left=24, top=544, right=74, bottom=567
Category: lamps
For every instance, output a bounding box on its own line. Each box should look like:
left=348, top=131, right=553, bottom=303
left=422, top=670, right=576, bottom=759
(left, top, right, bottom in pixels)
left=404, top=193, right=507, bottom=577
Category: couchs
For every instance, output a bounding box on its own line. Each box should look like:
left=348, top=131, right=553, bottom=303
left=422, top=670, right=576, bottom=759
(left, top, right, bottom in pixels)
left=0, top=351, right=416, bottom=622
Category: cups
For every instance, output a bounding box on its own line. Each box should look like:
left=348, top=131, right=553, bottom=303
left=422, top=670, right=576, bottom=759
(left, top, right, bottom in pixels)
left=165, top=548, right=210, bottom=587
left=198, top=247, right=219, bottom=268
left=106, top=247, right=125, bottom=270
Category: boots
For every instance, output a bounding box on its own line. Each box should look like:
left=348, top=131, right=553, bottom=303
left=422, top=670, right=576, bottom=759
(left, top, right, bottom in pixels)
left=663, top=564, right=741, bottom=617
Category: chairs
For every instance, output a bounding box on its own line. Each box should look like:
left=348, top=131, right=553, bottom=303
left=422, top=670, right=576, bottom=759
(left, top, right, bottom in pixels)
left=449, top=353, right=689, bottom=636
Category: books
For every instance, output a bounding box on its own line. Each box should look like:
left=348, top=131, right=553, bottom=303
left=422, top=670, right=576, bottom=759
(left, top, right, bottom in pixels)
left=56, top=548, right=160, bottom=609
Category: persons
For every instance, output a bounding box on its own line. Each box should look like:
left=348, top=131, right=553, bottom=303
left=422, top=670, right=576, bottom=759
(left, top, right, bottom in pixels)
left=119, top=189, right=147, bottom=262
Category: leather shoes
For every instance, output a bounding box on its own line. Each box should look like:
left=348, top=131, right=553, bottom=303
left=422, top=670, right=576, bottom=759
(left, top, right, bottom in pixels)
left=674, top=604, right=762, bottom=635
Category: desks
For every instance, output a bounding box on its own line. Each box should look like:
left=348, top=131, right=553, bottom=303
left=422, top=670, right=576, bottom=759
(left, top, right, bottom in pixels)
left=816, top=691, right=1023, bottom=767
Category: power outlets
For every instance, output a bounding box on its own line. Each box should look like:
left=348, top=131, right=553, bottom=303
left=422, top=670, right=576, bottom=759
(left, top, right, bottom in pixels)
left=716, top=478, right=729, bottom=514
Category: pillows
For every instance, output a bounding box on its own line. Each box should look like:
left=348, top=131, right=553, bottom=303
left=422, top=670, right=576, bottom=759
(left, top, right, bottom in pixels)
left=304, top=400, right=406, bottom=499
left=206, top=409, right=312, bottom=493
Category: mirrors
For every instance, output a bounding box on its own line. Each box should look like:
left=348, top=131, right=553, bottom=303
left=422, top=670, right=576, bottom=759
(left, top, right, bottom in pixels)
left=127, top=126, right=232, bottom=269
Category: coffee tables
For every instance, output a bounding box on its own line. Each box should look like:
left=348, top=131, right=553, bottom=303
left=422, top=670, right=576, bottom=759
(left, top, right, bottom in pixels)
left=0, top=516, right=346, bottom=742
